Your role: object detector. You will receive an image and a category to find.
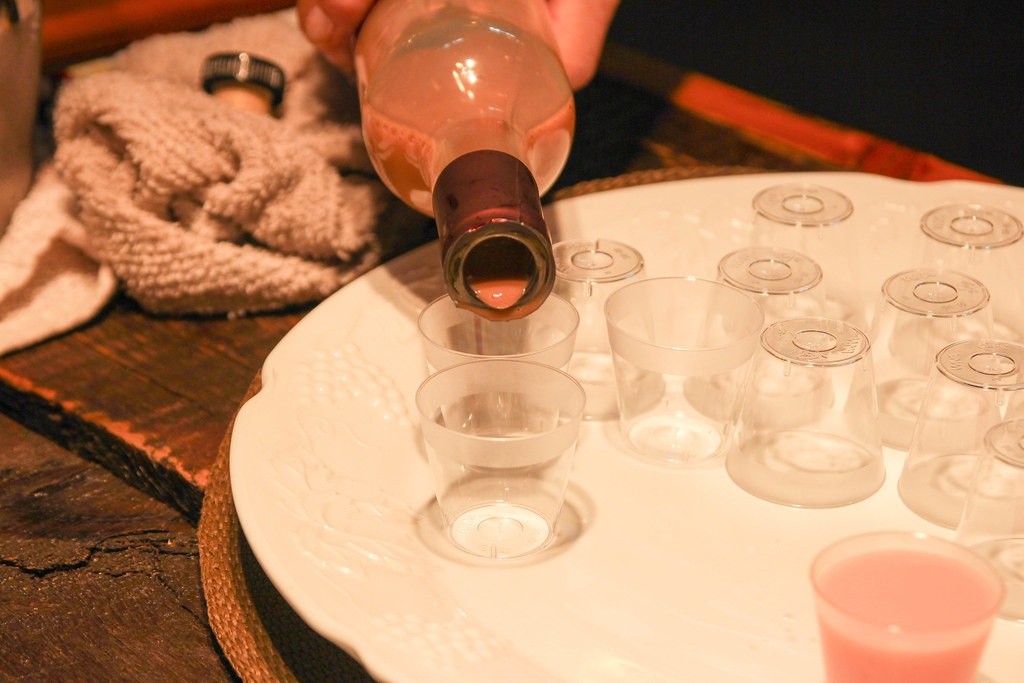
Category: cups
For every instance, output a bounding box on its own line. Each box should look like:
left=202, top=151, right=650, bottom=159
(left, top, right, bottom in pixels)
left=748, top=183, right=854, bottom=318
left=415, top=360, right=586, bottom=559
left=604, top=276, right=765, bottom=465
left=809, top=532, right=1007, bottom=683
left=844, top=268, right=993, bottom=452
left=523, top=238, right=667, bottom=421
left=956, top=418, right=1024, bottom=617
left=0, top=0, right=41, bottom=240
left=683, top=248, right=824, bottom=422
left=417, top=290, right=580, bottom=472
left=725, top=318, right=886, bottom=508
left=897, top=339, right=1024, bottom=531
left=890, top=203, right=1024, bottom=374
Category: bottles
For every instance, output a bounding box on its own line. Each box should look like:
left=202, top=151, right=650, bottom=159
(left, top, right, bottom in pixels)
left=354, top=0, right=575, bottom=320
